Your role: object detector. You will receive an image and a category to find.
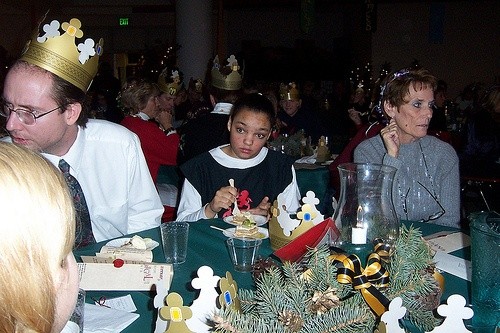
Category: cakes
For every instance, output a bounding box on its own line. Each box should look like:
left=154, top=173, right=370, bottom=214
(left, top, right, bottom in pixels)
left=232, top=210, right=260, bottom=237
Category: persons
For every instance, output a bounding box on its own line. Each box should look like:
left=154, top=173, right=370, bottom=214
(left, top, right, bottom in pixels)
left=175, top=94, right=301, bottom=223
left=354, top=69, right=460, bottom=229
left=0, top=141, right=81, bottom=333
left=0, top=10, right=164, bottom=249
left=91, top=54, right=500, bottom=222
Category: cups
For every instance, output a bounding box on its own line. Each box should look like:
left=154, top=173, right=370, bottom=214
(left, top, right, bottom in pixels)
left=227, top=236, right=262, bottom=272
left=160, top=221, right=189, bottom=264
left=469, top=218, right=500, bottom=332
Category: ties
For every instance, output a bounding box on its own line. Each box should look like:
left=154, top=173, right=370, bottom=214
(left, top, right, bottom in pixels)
left=58, top=160, right=97, bottom=246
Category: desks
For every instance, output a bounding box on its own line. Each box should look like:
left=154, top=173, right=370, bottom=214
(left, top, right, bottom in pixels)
left=296, top=161, right=335, bottom=218
left=72, top=217, right=473, bottom=333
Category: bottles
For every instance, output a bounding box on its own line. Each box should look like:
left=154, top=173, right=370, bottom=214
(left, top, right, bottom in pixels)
left=329, top=162, right=398, bottom=272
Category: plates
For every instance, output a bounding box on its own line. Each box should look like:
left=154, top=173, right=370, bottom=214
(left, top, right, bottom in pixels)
left=224, top=214, right=267, bottom=226
left=222, top=226, right=269, bottom=241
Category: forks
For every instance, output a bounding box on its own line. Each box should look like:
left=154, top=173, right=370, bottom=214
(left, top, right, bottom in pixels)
left=229, top=179, right=241, bottom=216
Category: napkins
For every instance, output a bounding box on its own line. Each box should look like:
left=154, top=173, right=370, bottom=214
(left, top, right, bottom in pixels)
left=295, top=156, right=316, bottom=164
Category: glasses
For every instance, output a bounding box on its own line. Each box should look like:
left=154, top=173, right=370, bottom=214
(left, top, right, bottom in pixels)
left=0, top=101, right=73, bottom=126
left=404, top=180, right=446, bottom=223
